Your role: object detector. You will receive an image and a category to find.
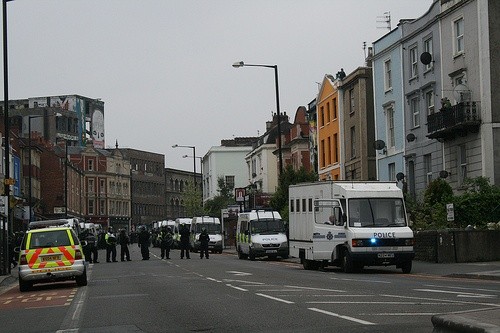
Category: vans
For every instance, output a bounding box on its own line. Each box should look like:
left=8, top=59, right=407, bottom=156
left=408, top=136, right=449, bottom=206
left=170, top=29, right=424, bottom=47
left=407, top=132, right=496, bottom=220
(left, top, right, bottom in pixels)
left=150, top=214, right=225, bottom=254
left=235, top=209, right=289, bottom=261
left=13, top=217, right=104, bottom=292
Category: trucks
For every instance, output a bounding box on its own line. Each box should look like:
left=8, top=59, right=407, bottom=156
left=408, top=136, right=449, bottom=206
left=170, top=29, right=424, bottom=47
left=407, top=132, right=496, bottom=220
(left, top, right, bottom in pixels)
left=287, top=180, right=415, bottom=274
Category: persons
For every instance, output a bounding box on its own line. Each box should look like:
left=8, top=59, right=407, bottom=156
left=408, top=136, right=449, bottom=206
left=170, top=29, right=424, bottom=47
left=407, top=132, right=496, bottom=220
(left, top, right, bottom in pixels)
left=86, top=232, right=99, bottom=263
left=319, top=222, right=330, bottom=272
left=179, top=223, right=191, bottom=259
left=104, top=226, right=119, bottom=263
left=157, top=227, right=174, bottom=250
left=158, top=226, right=171, bottom=259
left=139, top=227, right=150, bottom=260
left=120, top=230, right=131, bottom=261
left=329, top=216, right=341, bottom=263
left=199, top=227, right=210, bottom=259
left=152, top=228, right=157, bottom=248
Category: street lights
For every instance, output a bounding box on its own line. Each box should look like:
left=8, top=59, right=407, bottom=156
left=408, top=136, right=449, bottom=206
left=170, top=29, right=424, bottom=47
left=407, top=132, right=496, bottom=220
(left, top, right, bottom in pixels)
left=183, top=155, right=204, bottom=219
left=28, top=112, right=62, bottom=220
left=64, top=138, right=80, bottom=220
left=171, top=144, right=197, bottom=217
left=231, top=59, right=283, bottom=214
